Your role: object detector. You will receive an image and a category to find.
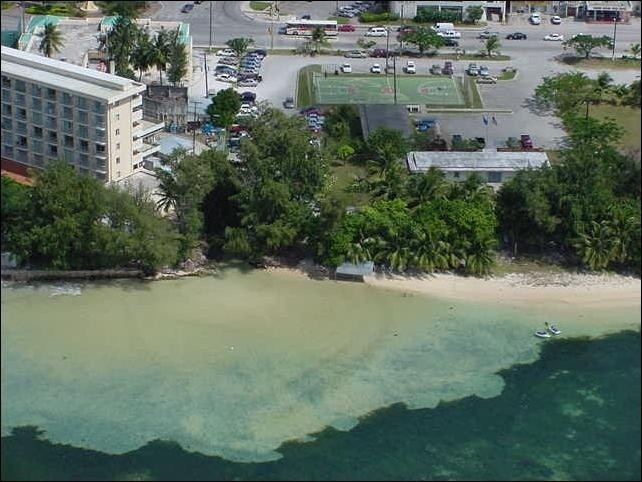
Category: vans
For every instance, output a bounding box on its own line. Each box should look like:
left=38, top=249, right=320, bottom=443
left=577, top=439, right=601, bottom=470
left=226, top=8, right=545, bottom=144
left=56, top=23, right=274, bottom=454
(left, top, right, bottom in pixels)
left=431, top=23, right=455, bottom=32
left=364, top=28, right=387, bottom=37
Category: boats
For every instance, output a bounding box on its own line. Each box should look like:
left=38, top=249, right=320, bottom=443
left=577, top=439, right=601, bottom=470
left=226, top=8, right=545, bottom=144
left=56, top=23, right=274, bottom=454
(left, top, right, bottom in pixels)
left=533, top=323, right=564, bottom=338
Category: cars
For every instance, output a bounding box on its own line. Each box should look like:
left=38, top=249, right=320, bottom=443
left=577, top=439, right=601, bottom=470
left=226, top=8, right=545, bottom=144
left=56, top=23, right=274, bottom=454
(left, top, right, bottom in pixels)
left=336, top=1, right=368, bottom=18
left=479, top=31, right=497, bottom=39
left=215, top=49, right=325, bottom=150
left=529, top=14, right=541, bottom=25
left=340, top=64, right=351, bottom=74
left=551, top=16, right=560, bottom=25
left=402, top=59, right=489, bottom=76
left=544, top=33, right=563, bottom=41
left=371, top=64, right=381, bottom=73
left=505, top=33, right=527, bottom=40
left=520, top=135, right=532, bottom=148
left=436, top=31, right=460, bottom=39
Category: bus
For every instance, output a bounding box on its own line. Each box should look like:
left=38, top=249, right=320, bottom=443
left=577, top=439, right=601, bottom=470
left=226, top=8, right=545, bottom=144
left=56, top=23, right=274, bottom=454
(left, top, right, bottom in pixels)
left=284, top=21, right=338, bottom=37
